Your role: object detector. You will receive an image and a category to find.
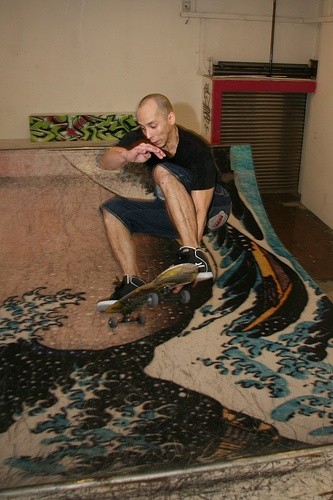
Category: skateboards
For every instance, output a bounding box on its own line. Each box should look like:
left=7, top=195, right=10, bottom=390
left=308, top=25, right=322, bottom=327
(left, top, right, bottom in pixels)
left=100, top=263, right=197, bottom=329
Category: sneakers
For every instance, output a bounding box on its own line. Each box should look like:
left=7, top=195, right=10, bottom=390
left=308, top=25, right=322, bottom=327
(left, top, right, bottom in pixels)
left=170, top=246, right=214, bottom=282
left=96, top=275, right=148, bottom=312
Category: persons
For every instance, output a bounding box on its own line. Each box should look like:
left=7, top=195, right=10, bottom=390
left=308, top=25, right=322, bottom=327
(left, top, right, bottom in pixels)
left=95, top=92, right=226, bottom=315
